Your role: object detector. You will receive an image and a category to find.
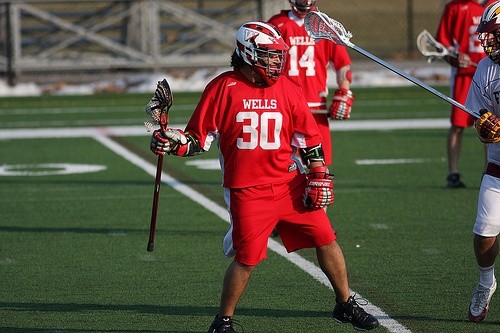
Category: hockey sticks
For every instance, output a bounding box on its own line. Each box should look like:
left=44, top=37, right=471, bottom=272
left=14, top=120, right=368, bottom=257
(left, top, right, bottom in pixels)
left=144, top=78, right=174, bottom=253
left=417, top=31, right=478, bottom=68
left=304, top=12, right=481, bottom=118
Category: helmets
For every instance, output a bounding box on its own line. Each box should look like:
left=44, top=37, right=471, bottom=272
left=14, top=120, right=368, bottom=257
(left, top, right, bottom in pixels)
left=288, top=0, right=320, bottom=19
left=235, top=21, right=291, bottom=85
left=476, top=0, right=500, bottom=64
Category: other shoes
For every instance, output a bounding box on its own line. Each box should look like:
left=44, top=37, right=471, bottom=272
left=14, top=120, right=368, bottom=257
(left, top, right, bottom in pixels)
left=446, top=173, right=464, bottom=188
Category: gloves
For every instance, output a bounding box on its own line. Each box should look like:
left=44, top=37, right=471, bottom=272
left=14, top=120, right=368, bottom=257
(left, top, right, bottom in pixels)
left=475, top=109, right=500, bottom=144
left=445, top=49, right=471, bottom=69
left=328, top=88, right=355, bottom=121
left=150, top=127, right=189, bottom=156
left=303, top=166, right=335, bottom=209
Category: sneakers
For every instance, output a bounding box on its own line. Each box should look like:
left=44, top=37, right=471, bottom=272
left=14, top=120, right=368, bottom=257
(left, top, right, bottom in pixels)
left=333, top=293, right=379, bottom=330
left=207, top=314, right=244, bottom=333
left=469, top=276, right=498, bottom=323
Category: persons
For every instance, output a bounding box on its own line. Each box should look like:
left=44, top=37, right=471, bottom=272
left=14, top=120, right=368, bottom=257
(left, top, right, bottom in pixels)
left=268, top=0, right=354, bottom=239
left=437, top=0, right=500, bottom=188
left=149, top=21, right=379, bottom=333
left=464, top=0, right=500, bottom=322
